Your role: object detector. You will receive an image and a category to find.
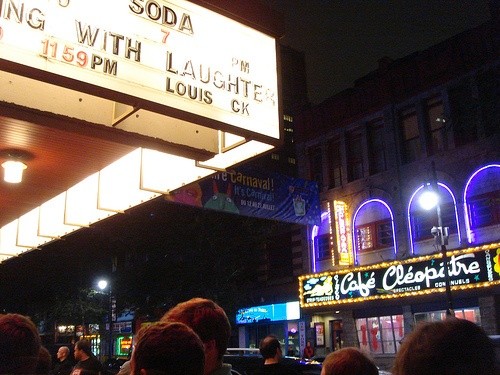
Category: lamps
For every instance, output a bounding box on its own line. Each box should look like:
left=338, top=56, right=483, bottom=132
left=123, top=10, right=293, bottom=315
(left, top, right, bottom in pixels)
left=1, top=154, right=26, bottom=184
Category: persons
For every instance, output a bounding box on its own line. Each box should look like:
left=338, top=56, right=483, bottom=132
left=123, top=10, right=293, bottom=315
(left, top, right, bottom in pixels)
left=0, top=313, right=42, bottom=375
left=52, top=346, right=73, bottom=375
left=304, top=342, right=314, bottom=358
left=391, top=317, right=499, bottom=375
left=70, top=340, right=104, bottom=375
left=129, top=321, right=207, bottom=375
left=320, top=347, right=379, bottom=375
left=159, top=297, right=232, bottom=375
left=252, top=335, right=303, bottom=375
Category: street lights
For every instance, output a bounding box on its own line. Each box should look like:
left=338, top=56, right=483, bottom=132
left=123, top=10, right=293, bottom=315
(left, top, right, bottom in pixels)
left=97, top=280, right=112, bottom=358
left=419, top=176, right=453, bottom=319
left=3, top=159, right=28, bottom=187
